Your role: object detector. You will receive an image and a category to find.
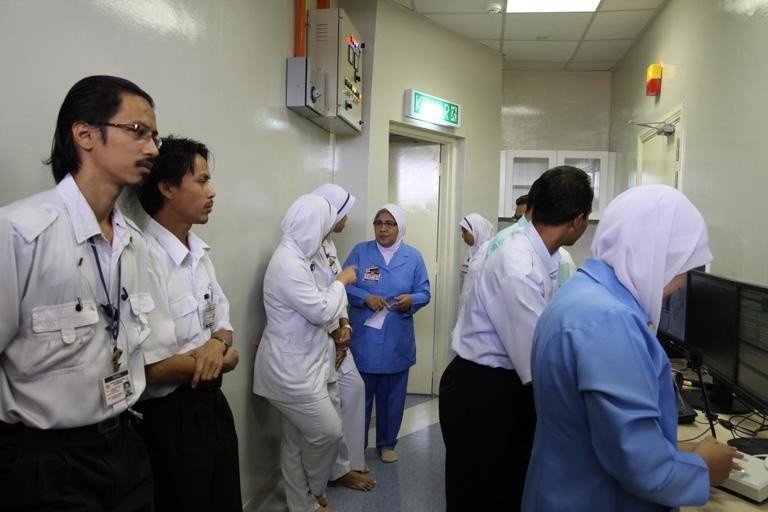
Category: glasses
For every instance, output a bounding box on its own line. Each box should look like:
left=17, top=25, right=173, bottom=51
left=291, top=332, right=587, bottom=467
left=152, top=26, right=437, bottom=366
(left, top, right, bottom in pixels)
left=373, top=221, right=398, bottom=229
left=103, top=119, right=165, bottom=149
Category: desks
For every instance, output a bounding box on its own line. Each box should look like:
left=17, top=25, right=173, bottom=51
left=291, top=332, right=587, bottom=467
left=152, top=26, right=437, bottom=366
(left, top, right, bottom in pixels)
left=677, top=356, right=767, bottom=510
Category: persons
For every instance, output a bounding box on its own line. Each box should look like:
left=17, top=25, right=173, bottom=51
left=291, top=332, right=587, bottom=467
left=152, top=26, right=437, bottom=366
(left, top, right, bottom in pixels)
left=457, top=166, right=592, bottom=343
left=0, top=75, right=161, bottom=510
left=438, top=171, right=594, bottom=512
left=519, top=182, right=743, bottom=512
left=137, top=134, right=246, bottom=512
left=315, top=182, right=377, bottom=493
left=123, top=381, right=132, bottom=398
left=341, top=202, right=432, bottom=474
left=255, top=195, right=361, bottom=512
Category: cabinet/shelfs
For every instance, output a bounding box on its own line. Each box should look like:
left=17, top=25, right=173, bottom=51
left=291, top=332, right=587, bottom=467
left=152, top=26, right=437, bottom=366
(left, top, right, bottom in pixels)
left=497, top=150, right=614, bottom=220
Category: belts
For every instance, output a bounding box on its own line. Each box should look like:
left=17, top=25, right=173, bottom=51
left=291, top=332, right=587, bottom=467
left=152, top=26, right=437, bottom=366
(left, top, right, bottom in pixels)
left=62, top=410, right=133, bottom=435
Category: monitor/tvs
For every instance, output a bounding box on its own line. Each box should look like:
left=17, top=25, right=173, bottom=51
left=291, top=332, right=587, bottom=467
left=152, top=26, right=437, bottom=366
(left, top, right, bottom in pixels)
left=657, top=261, right=768, bottom=418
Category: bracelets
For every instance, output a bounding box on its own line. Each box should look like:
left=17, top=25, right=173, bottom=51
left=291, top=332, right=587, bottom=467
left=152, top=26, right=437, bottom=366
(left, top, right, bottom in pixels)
left=343, top=323, right=352, bottom=332
left=212, top=334, right=229, bottom=354
